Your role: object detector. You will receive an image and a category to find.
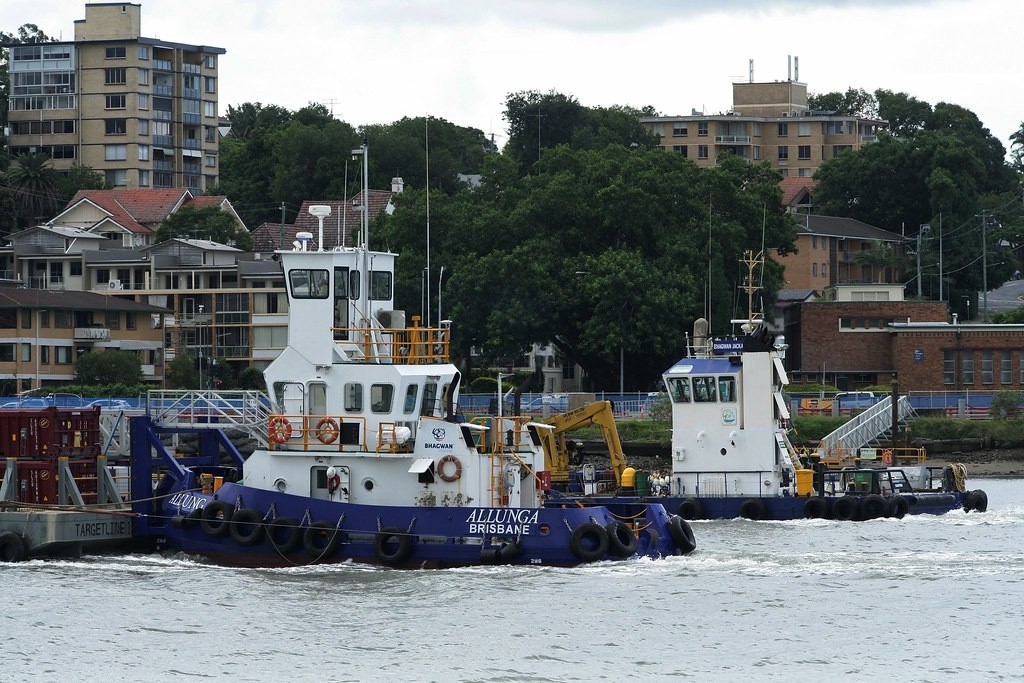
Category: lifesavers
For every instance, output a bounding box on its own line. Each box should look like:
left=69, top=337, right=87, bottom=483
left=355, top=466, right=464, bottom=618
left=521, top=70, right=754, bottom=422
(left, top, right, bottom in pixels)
left=831, top=496, right=858, bottom=520
left=0, top=530, right=26, bottom=562
left=571, top=522, right=608, bottom=561
left=304, top=519, right=342, bottom=557
left=315, top=419, right=339, bottom=445
left=201, top=500, right=233, bottom=536
left=884, top=452, right=893, bottom=464
left=609, top=521, right=639, bottom=559
left=860, top=494, right=887, bottom=521
left=665, top=514, right=697, bottom=552
left=739, top=499, right=762, bottom=520
left=438, top=454, right=462, bottom=482
left=373, top=528, right=416, bottom=566
left=804, top=497, right=829, bottom=520
left=264, top=517, right=299, bottom=552
left=267, top=418, right=293, bottom=442
left=678, top=500, right=700, bottom=519
left=887, top=494, right=909, bottom=519
left=229, top=510, right=264, bottom=547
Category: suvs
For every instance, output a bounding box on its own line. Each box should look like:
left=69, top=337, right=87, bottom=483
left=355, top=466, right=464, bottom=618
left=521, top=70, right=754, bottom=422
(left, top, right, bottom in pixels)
left=47, top=392, right=90, bottom=406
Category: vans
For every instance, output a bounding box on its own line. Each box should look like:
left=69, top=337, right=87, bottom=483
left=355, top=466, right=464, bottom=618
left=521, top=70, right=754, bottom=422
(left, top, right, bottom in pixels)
left=829, top=392, right=875, bottom=408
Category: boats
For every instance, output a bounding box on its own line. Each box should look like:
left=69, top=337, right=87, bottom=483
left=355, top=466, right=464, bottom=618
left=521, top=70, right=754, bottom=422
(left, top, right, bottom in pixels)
left=127, top=146, right=697, bottom=571
left=547, top=255, right=989, bottom=521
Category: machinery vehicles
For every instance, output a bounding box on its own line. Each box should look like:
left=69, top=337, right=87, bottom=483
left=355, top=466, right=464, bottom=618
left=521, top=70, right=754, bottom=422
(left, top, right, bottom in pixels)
left=488, top=401, right=628, bottom=489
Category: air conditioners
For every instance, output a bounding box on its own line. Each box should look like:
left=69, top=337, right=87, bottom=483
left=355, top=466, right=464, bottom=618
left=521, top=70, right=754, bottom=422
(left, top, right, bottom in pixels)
left=733, top=113, right=742, bottom=117
left=109, top=279, right=121, bottom=292
left=378, top=309, right=406, bottom=331
left=782, top=111, right=790, bottom=117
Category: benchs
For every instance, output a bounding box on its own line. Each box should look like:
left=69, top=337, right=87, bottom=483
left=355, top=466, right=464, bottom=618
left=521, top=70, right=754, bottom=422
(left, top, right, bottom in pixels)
left=883, top=465, right=926, bottom=490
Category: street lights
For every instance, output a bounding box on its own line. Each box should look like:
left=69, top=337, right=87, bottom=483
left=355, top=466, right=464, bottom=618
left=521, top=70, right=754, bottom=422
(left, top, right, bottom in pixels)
left=198, top=305, right=204, bottom=400
left=983, top=262, right=1005, bottom=321
left=914, top=263, right=940, bottom=299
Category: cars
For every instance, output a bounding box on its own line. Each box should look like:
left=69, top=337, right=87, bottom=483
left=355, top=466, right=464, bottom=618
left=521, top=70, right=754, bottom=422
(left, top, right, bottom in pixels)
left=2, top=399, right=185, bottom=409
